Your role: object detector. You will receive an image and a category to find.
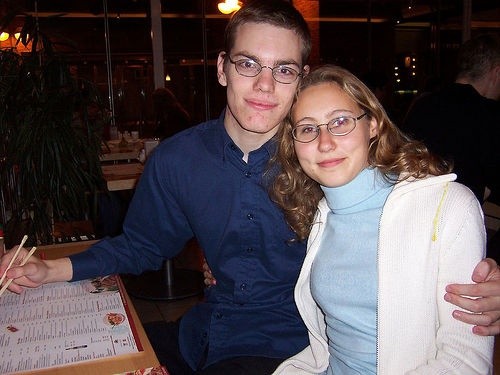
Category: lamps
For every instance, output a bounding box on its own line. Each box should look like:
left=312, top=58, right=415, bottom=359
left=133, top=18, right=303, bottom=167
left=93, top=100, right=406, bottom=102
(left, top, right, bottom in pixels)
left=15, top=28, right=29, bottom=42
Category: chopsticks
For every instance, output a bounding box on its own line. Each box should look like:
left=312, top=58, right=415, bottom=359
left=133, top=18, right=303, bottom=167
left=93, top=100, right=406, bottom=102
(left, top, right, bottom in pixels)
left=0, top=234, right=37, bottom=297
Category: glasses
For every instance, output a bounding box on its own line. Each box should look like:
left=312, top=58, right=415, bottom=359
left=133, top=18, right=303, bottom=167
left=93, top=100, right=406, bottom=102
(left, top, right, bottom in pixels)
left=226, top=52, right=303, bottom=84
left=288, top=112, right=368, bottom=143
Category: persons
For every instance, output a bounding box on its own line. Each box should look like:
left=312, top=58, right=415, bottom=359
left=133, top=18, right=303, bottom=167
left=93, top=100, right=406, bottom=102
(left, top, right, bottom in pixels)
left=151, top=88, right=192, bottom=139
left=400, top=31, right=499, bottom=206
left=0, top=1, right=500, bottom=375
left=203, top=65, right=494, bottom=375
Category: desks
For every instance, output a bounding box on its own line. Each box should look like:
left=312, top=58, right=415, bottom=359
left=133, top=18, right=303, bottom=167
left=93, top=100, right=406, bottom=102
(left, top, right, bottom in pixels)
left=98, top=138, right=208, bottom=301
left=0, top=239, right=168, bottom=375
